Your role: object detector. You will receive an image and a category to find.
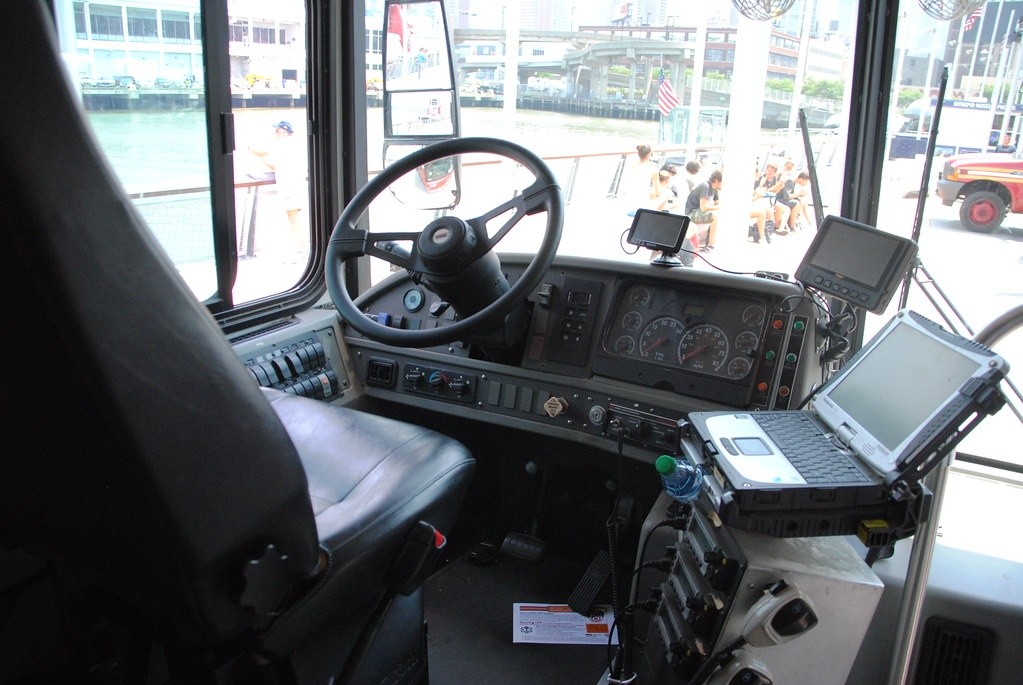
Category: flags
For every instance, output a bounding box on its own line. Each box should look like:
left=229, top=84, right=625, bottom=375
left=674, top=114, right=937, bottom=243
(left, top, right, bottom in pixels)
left=657, top=69, right=680, bottom=117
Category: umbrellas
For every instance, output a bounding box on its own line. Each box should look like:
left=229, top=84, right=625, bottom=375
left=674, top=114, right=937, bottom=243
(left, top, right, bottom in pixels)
left=824, top=114, right=841, bottom=125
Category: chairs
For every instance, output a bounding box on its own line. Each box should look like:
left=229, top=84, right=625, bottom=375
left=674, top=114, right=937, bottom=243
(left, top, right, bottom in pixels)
left=0, top=0, right=477, bottom=685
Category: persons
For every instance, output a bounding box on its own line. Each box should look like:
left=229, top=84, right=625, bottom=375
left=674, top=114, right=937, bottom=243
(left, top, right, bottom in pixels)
left=419, top=48, right=427, bottom=80
left=624, top=144, right=722, bottom=267
left=995, top=135, right=1016, bottom=153
left=829, top=127, right=839, bottom=135
left=247, top=121, right=305, bottom=262
left=751, top=162, right=811, bottom=244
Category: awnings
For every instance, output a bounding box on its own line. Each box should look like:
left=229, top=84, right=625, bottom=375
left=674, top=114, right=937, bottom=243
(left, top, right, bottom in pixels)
left=905, top=99, right=931, bottom=116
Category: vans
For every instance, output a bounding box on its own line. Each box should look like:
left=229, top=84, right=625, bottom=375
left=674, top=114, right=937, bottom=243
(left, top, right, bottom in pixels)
left=893, top=98, right=1022, bottom=157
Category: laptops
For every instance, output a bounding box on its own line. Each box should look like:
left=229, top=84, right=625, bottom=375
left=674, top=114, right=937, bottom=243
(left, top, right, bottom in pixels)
left=688, top=308, right=1009, bottom=512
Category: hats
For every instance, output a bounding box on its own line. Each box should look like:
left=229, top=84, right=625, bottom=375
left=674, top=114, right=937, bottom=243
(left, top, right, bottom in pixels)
left=273, top=121, right=293, bottom=134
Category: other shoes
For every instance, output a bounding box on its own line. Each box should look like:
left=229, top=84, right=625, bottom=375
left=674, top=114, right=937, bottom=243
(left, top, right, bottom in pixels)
left=775, top=229, right=788, bottom=236
left=699, top=246, right=710, bottom=253
left=706, top=243, right=715, bottom=249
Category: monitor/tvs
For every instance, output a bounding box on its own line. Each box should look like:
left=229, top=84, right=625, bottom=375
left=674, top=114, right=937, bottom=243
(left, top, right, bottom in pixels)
left=626, top=209, right=691, bottom=253
left=795, top=214, right=919, bottom=315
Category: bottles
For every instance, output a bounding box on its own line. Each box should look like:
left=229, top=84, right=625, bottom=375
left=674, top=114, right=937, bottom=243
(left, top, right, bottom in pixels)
left=655, top=455, right=707, bottom=503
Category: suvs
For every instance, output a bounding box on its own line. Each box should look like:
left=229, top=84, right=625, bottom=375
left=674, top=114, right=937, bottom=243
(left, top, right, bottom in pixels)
left=936, top=144, right=1023, bottom=233
